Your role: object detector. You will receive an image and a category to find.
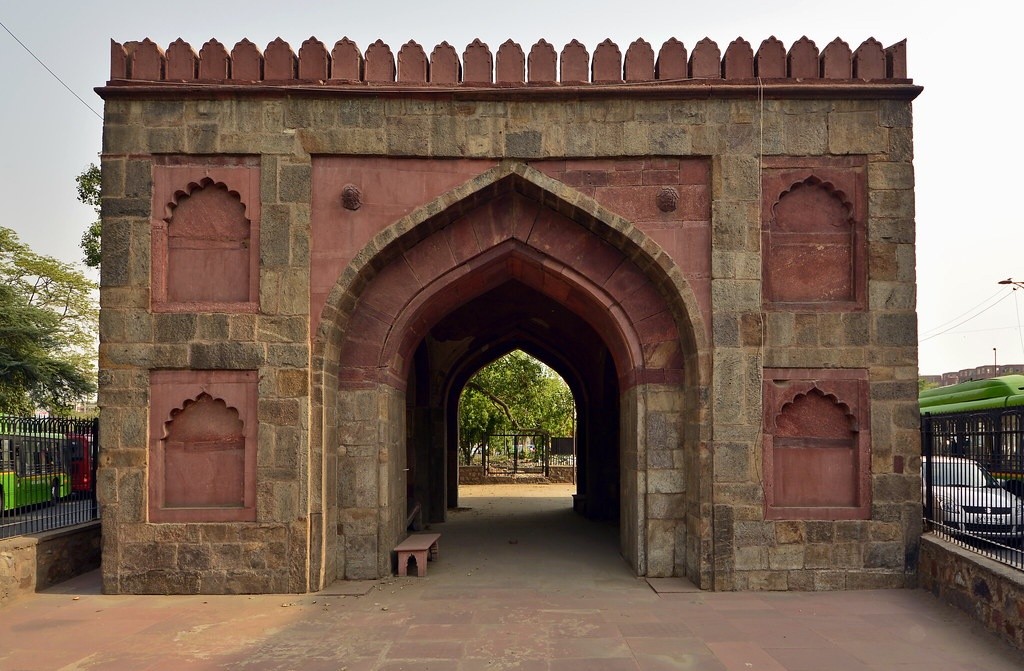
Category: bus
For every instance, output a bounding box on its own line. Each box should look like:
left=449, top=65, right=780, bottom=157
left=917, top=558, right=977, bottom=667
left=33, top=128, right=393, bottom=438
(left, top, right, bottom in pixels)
left=919, top=374, right=1024, bottom=498
left=0, top=413, right=94, bottom=516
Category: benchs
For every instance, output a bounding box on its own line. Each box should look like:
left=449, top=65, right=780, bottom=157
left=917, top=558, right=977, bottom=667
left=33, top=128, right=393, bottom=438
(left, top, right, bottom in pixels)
left=572, top=495, right=588, bottom=516
left=393, top=534, right=442, bottom=576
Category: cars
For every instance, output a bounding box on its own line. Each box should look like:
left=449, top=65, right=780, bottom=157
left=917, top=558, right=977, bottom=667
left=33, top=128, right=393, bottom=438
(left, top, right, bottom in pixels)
left=921, top=456, right=1024, bottom=546
